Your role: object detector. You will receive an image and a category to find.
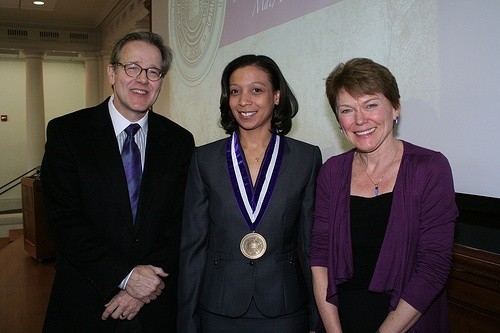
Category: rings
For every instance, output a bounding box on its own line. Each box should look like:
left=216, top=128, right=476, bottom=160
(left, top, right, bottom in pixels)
left=121, top=313, right=126, bottom=318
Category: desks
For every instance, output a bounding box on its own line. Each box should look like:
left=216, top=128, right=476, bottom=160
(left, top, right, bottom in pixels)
left=444, top=223, right=500, bottom=332
left=20, top=176, right=56, bottom=264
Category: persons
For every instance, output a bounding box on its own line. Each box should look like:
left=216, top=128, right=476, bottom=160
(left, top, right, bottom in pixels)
left=169, top=54, right=323, bottom=333
left=309, top=58, right=459, bottom=333
left=34, top=32, right=194, bottom=333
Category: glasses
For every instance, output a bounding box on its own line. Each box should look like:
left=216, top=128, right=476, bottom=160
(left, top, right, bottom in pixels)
left=111, top=62, right=163, bottom=81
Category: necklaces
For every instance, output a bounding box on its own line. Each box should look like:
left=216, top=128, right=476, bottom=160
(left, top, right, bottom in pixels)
left=358, top=137, right=399, bottom=196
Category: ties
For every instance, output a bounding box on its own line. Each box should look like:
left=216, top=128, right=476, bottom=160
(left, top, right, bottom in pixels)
left=120, top=123, right=142, bottom=225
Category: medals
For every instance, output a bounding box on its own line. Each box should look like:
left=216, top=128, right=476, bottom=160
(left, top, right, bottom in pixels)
left=240, top=232, right=267, bottom=259
